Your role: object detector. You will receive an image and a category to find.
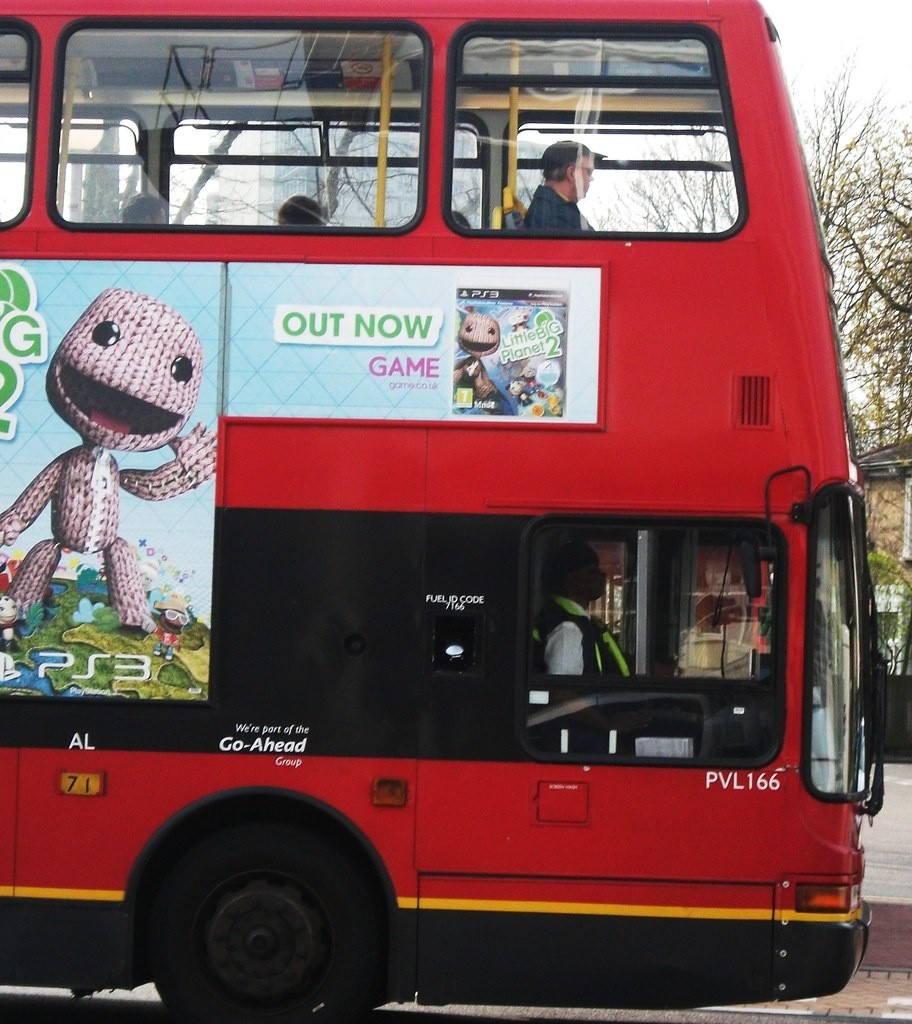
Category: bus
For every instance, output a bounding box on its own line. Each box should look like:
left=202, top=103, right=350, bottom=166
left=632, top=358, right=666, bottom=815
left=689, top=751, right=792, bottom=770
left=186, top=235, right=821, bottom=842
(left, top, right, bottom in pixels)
left=1, top=0, right=897, bottom=1024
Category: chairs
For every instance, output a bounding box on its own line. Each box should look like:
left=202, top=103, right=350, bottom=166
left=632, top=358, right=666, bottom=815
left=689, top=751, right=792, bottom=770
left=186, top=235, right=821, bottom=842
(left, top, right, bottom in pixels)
left=490, top=187, right=530, bottom=230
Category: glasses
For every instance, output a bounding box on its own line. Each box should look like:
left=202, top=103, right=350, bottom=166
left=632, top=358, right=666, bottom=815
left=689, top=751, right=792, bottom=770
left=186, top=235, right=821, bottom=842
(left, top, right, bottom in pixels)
left=575, top=166, right=594, bottom=177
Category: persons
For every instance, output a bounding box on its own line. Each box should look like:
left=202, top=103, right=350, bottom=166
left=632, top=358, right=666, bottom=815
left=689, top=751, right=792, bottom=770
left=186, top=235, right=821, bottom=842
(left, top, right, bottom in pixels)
left=277, top=196, right=321, bottom=226
left=535, top=543, right=631, bottom=675
left=525, top=141, right=594, bottom=230
left=121, top=194, right=167, bottom=223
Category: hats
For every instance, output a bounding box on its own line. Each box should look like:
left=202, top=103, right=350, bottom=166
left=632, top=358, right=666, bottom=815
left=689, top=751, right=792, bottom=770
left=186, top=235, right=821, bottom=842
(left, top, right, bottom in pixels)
left=540, top=140, right=608, bottom=168
